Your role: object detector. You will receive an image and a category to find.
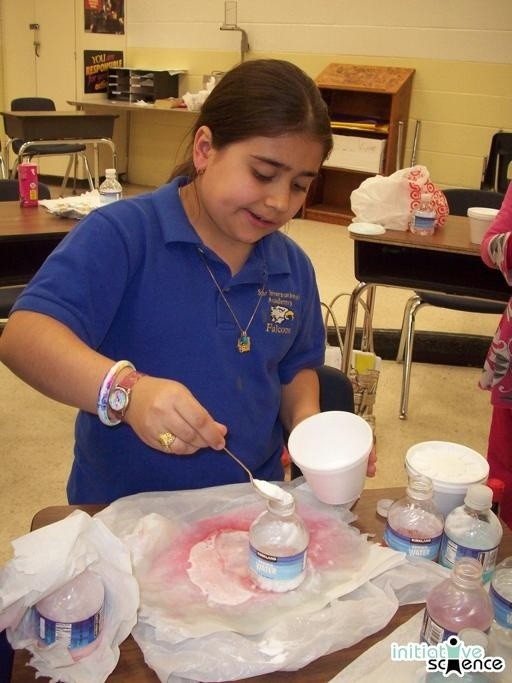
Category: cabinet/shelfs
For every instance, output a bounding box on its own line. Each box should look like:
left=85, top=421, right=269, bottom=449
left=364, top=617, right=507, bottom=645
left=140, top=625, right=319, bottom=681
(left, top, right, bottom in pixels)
left=107, top=66, right=183, bottom=102
left=299, top=62, right=415, bottom=226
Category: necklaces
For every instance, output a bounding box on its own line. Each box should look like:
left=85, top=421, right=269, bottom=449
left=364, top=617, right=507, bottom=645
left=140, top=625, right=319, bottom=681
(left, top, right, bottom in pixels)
left=195, top=244, right=269, bottom=355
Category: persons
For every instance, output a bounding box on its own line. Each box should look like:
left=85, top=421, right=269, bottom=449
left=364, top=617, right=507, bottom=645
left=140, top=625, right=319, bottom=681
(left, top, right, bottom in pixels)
left=478, top=177, right=511, bottom=532
left=1, top=56, right=336, bottom=505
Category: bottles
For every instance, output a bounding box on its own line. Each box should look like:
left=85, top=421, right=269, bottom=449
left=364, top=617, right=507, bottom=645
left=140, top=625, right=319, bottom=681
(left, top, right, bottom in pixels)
left=380, top=474, right=512, bottom=683
left=247, top=498, right=308, bottom=594
left=31, top=572, right=109, bottom=658
left=409, top=192, right=439, bottom=236
left=97, top=168, right=125, bottom=203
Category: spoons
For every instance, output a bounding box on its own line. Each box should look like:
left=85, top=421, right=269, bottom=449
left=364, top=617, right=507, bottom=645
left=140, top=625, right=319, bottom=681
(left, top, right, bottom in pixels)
left=219, top=446, right=294, bottom=503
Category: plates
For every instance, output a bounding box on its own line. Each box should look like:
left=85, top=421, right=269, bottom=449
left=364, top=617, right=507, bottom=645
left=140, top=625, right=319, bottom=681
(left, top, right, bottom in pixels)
left=348, top=221, right=386, bottom=235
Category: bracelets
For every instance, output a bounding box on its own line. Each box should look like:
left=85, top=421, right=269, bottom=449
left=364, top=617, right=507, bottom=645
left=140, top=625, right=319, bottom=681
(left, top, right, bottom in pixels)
left=96, top=359, right=137, bottom=427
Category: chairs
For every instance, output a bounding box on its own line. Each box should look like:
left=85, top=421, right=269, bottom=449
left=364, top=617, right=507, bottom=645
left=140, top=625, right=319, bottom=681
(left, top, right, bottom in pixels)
left=395, top=186, right=511, bottom=419
left=7, top=97, right=95, bottom=197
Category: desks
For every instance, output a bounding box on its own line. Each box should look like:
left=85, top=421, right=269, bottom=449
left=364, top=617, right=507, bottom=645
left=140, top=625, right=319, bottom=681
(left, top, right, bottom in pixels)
left=339, top=211, right=512, bottom=384
left=0, top=109, right=120, bottom=186
left=65, top=99, right=200, bottom=186
left=0, top=196, right=99, bottom=319
left=12, top=480, right=512, bottom=682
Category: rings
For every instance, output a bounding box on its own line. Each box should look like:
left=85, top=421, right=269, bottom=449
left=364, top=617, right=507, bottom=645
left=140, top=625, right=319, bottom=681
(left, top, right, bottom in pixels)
left=160, top=432, right=178, bottom=454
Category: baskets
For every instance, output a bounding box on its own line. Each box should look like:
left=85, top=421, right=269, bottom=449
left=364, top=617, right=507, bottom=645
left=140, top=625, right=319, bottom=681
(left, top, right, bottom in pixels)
left=319, top=294, right=380, bottom=443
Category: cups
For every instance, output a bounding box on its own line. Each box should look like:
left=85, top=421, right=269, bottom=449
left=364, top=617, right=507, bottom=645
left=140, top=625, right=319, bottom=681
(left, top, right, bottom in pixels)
left=403, top=440, right=491, bottom=514
left=286, top=408, right=377, bottom=508
left=467, top=206, right=500, bottom=246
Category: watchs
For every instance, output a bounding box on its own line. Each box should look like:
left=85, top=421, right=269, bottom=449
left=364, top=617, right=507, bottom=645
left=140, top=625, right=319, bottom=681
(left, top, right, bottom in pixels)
left=108, top=370, right=150, bottom=423
